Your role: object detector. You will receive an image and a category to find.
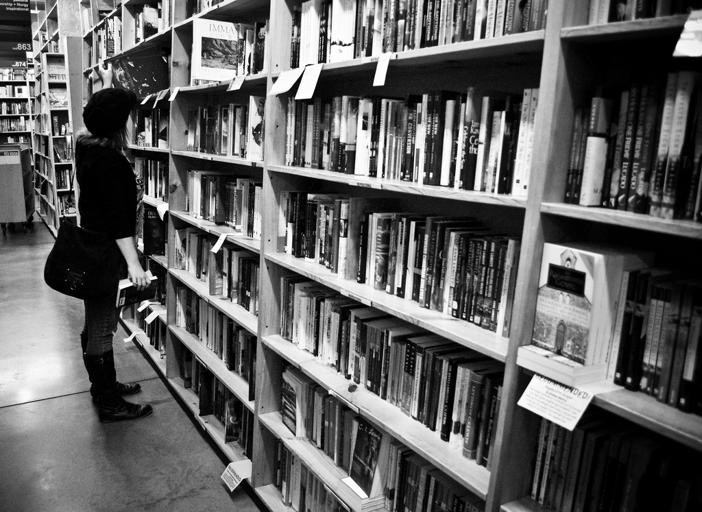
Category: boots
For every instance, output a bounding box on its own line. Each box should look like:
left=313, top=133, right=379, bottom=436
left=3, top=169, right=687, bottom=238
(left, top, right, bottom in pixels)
left=80, top=347, right=149, bottom=424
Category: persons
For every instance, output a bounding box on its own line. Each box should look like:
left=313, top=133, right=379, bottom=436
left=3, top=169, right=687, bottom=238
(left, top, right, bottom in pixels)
left=76, top=86, right=153, bottom=422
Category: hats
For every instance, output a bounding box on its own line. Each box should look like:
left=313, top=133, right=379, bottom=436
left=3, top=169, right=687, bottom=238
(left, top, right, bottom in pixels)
left=82, top=87, right=137, bottom=136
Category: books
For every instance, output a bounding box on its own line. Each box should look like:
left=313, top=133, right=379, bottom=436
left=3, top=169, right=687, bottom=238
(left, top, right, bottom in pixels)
left=132, top=204, right=261, bottom=462
left=1, top=30, right=75, bottom=231
left=87, top=0, right=270, bottom=242
left=273, top=0, right=701, bottom=512
left=116, top=269, right=157, bottom=307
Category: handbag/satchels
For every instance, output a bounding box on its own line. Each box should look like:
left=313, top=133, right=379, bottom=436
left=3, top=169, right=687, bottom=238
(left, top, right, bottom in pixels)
left=44, top=218, right=90, bottom=300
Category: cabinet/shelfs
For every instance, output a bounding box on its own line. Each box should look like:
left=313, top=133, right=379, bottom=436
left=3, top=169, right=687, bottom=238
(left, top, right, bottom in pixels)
left=0, top=0, right=702, bottom=512
